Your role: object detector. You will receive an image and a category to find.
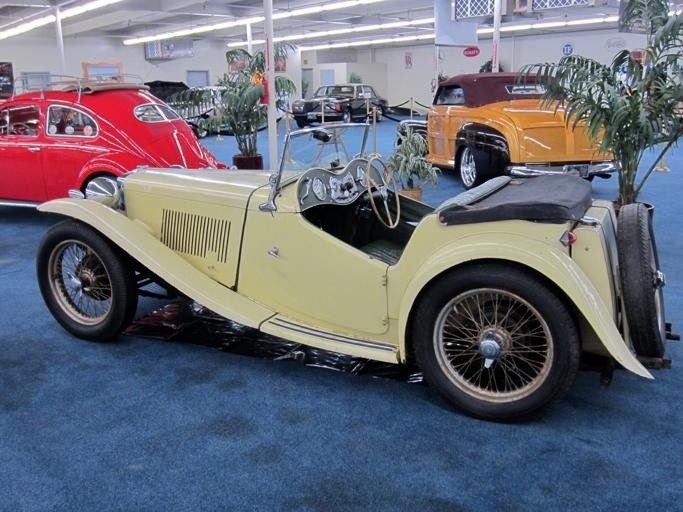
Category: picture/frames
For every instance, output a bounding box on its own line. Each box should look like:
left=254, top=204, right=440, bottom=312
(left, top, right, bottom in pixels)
left=81, top=60, right=125, bottom=83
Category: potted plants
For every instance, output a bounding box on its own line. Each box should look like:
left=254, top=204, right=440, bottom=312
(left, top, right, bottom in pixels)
left=383, top=125, right=442, bottom=203
left=165, top=40, right=298, bottom=170
left=514, top=0, right=683, bottom=224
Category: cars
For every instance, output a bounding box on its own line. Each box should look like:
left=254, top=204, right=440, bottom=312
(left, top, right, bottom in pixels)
left=0, top=74, right=240, bottom=209
left=141, top=85, right=259, bottom=139
left=290, top=83, right=388, bottom=128
left=393, top=71, right=628, bottom=194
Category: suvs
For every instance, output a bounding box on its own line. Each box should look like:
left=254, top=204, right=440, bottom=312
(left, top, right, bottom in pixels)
left=31, top=120, right=683, bottom=425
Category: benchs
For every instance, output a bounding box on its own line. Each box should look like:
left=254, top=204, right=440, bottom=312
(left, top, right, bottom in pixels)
left=357, top=175, right=512, bottom=267
